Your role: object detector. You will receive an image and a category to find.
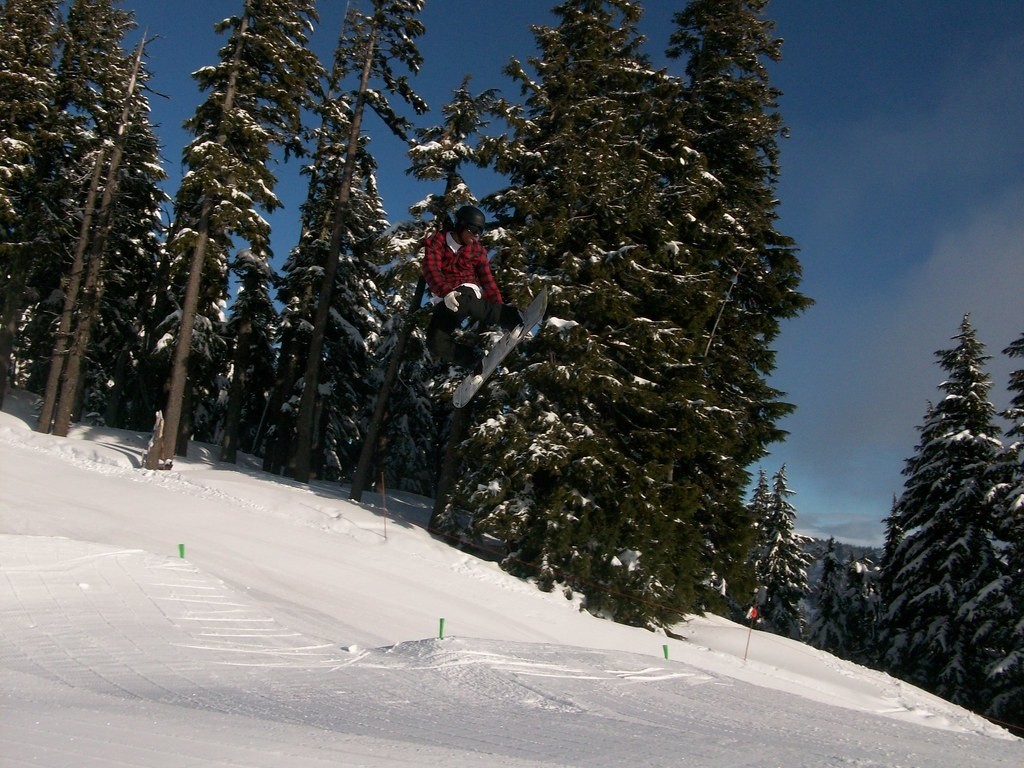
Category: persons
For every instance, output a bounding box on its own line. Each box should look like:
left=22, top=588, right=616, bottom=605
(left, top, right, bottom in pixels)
left=422, top=206, right=524, bottom=384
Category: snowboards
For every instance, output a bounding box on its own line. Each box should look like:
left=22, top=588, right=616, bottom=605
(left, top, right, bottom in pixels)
left=451, top=285, right=549, bottom=409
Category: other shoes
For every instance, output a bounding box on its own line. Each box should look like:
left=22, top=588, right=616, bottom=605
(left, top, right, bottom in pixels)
left=501, top=305, right=523, bottom=340
left=467, top=359, right=482, bottom=386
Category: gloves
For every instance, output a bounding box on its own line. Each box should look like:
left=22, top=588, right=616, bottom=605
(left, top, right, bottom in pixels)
left=444, top=291, right=461, bottom=312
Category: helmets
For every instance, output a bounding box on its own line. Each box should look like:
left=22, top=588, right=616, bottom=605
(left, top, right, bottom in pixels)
left=455, top=205, right=485, bottom=235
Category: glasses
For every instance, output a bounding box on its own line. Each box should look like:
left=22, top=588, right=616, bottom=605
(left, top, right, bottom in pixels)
left=465, top=225, right=481, bottom=237
